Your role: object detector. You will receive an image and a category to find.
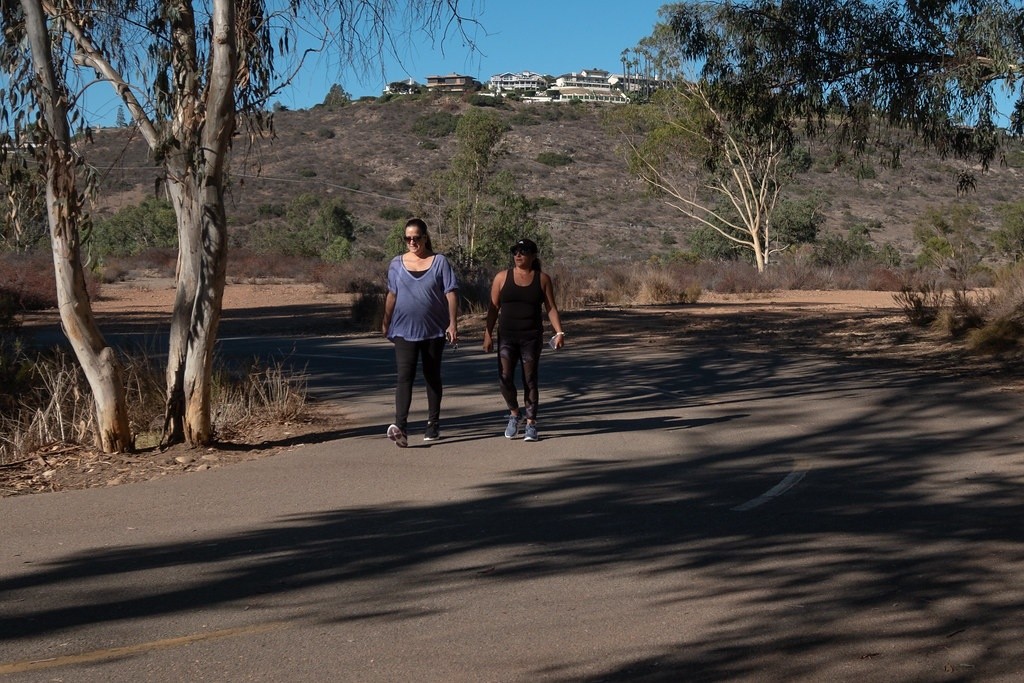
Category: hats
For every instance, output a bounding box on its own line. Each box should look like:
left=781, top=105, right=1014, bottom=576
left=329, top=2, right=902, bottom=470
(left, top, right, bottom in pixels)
left=510, top=238, right=538, bottom=253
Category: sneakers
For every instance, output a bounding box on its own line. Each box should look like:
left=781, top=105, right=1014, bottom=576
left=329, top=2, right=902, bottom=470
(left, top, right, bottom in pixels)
left=505, top=410, right=524, bottom=438
left=524, top=424, right=538, bottom=441
left=424, top=422, right=440, bottom=440
left=387, top=424, right=408, bottom=447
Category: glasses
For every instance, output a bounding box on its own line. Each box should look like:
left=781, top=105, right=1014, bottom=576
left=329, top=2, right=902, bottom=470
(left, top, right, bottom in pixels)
left=404, top=235, right=423, bottom=243
left=512, top=249, right=531, bottom=256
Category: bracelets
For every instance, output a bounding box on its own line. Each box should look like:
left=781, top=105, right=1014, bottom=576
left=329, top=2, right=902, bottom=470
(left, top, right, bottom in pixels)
left=556, top=332, right=565, bottom=336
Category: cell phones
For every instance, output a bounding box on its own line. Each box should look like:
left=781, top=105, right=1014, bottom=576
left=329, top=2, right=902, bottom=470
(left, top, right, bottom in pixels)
left=548, top=336, right=560, bottom=351
left=444, top=332, right=452, bottom=342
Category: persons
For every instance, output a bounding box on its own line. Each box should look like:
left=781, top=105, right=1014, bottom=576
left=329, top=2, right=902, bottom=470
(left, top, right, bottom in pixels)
left=382, top=219, right=459, bottom=448
left=483, top=239, right=565, bottom=441
